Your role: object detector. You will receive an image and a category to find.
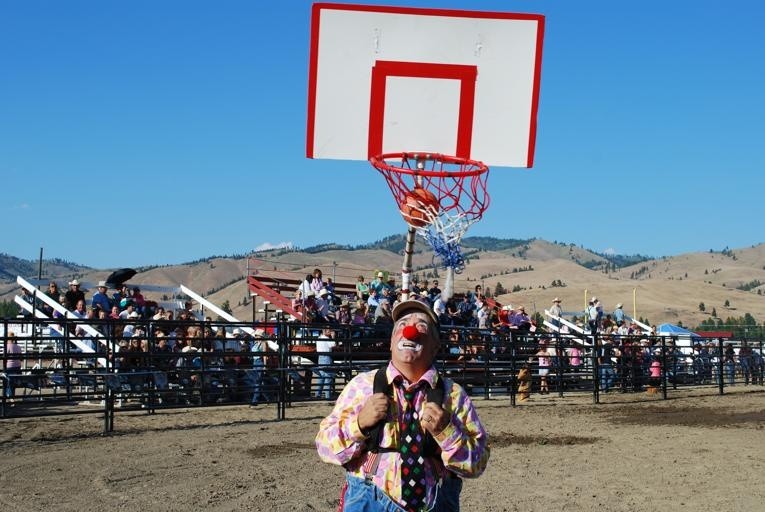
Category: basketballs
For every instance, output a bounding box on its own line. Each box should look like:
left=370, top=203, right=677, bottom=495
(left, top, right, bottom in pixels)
left=400, top=189, right=439, bottom=227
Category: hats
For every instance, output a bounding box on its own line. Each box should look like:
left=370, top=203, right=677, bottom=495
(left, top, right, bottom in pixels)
left=68, top=279, right=80, bottom=287
left=589, top=296, right=623, bottom=308
left=409, top=290, right=429, bottom=297
left=378, top=271, right=384, bottom=279
left=254, top=329, right=265, bottom=338
left=94, top=280, right=111, bottom=289
left=230, top=328, right=243, bottom=336
left=306, top=288, right=329, bottom=297
left=551, top=297, right=561, bottom=303
left=391, top=299, right=441, bottom=325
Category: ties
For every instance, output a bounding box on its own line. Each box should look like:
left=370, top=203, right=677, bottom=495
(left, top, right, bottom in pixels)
left=397, top=382, right=426, bottom=508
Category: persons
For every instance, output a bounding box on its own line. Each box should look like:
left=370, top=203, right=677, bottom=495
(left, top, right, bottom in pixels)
left=315, top=299, right=491, bottom=512
left=1, top=270, right=764, bottom=405
